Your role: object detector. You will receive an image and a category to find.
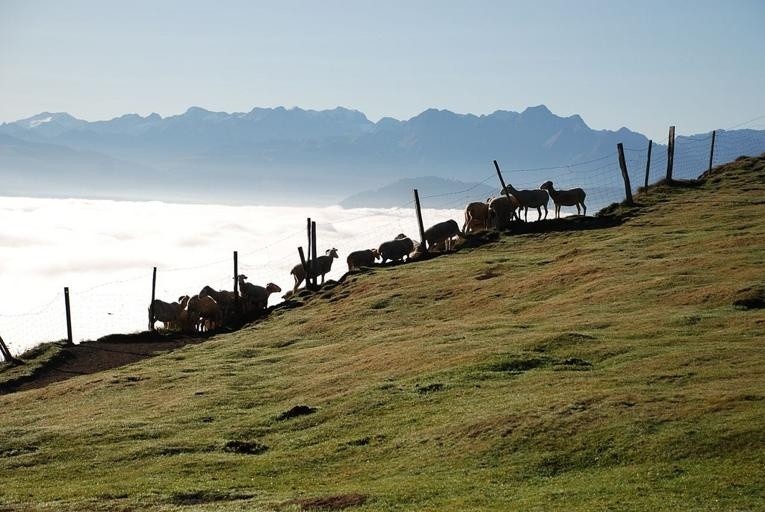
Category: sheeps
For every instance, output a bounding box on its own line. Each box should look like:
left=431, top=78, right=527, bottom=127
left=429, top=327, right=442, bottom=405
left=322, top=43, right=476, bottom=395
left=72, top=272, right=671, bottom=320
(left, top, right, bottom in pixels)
left=291, top=247, right=339, bottom=294
left=148, top=274, right=281, bottom=335
left=540, top=181, right=587, bottom=219
left=462, top=196, right=524, bottom=235
left=423, top=219, right=461, bottom=254
left=378, top=234, right=421, bottom=263
left=347, top=248, right=380, bottom=272
left=500, top=183, right=549, bottom=223
